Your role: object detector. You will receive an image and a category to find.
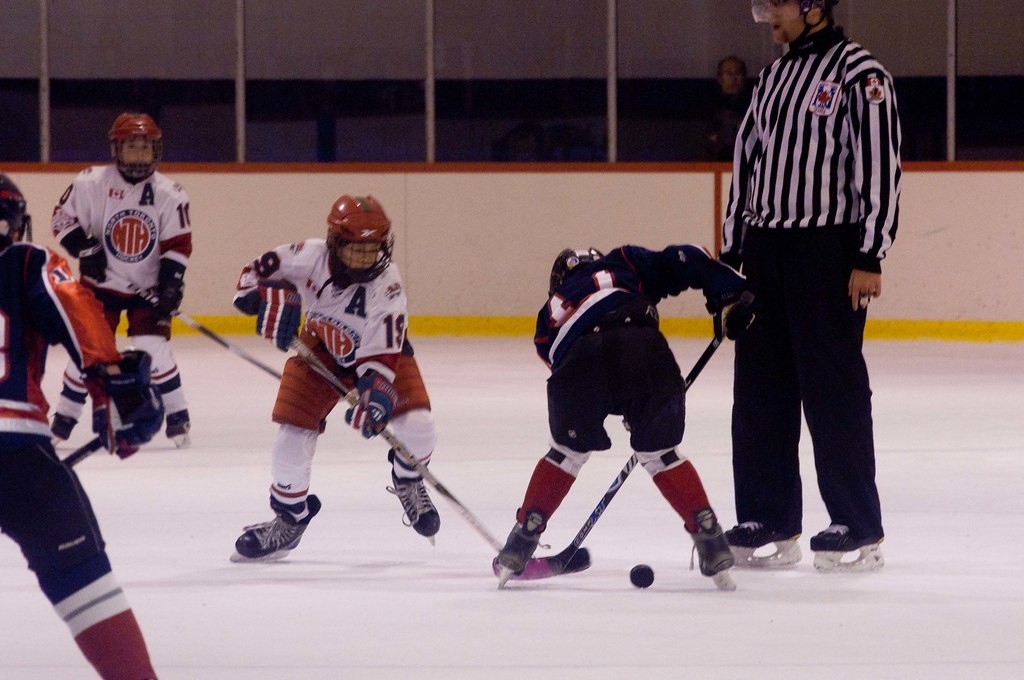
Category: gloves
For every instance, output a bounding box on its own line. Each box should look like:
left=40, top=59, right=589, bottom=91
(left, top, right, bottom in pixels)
left=156, top=272, right=184, bottom=315
left=80, top=350, right=164, bottom=459
left=79, top=237, right=107, bottom=283
left=345, top=370, right=397, bottom=437
left=256, top=285, right=301, bottom=352
left=707, top=295, right=756, bottom=340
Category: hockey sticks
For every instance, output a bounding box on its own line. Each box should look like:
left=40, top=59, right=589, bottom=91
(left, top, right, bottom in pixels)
left=291, top=334, right=591, bottom=582
left=101, top=265, right=329, bottom=436
left=61, top=398, right=163, bottom=468
left=490, top=288, right=757, bottom=581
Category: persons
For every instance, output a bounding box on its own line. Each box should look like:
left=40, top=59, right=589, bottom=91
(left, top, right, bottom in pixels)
left=50, top=113, right=193, bottom=449
left=494, top=243, right=745, bottom=592
left=0, top=173, right=166, bottom=680
left=230, top=195, right=437, bottom=559
left=705, top=55, right=748, bottom=158
left=717, top=0, right=902, bottom=574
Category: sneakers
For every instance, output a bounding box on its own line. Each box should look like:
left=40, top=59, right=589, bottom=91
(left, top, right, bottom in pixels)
left=231, top=495, right=321, bottom=562
left=166, top=418, right=191, bottom=447
left=498, top=508, right=547, bottom=588
left=811, top=524, right=885, bottom=570
left=685, top=508, right=736, bottom=590
left=725, top=520, right=802, bottom=566
left=388, top=448, right=440, bottom=545
left=51, top=412, right=77, bottom=446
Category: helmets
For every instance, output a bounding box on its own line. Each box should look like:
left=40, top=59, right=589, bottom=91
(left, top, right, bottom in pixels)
left=549, top=249, right=602, bottom=297
left=108, top=112, right=162, bottom=178
left=0, top=174, right=27, bottom=224
left=326, top=194, right=394, bottom=283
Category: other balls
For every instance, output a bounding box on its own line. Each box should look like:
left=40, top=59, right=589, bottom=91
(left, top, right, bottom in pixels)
left=629, top=564, right=655, bottom=588
